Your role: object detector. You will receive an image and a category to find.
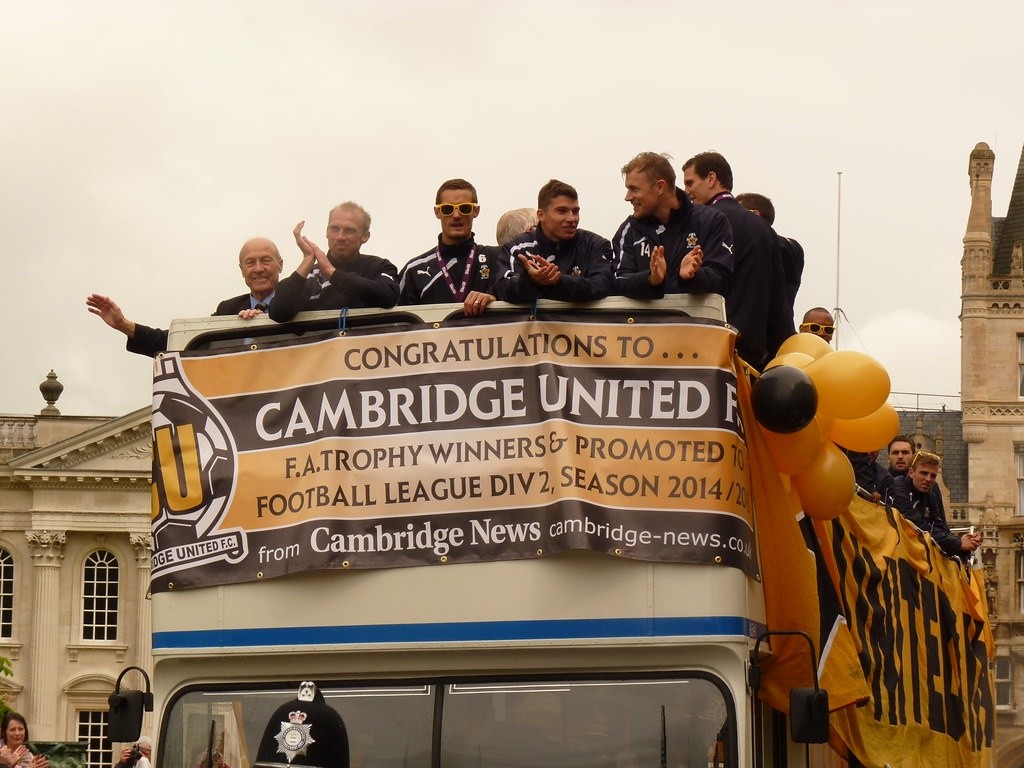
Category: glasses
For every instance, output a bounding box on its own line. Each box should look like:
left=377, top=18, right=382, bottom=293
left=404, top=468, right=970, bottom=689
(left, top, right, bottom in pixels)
left=802, top=323, right=836, bottom=336
left=435, top=202, right=477, bottom=216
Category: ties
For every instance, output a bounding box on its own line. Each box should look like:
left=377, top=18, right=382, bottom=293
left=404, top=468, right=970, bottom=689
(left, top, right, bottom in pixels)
left=255, top=302, right=267, bottom=312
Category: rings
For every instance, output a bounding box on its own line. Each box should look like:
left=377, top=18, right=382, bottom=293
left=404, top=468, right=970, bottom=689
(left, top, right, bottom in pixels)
left=474, top=301, right=479, bottom=304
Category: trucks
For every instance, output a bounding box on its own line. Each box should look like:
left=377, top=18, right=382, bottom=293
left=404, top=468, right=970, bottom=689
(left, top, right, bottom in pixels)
left=105, top=291, right=996, bottom=768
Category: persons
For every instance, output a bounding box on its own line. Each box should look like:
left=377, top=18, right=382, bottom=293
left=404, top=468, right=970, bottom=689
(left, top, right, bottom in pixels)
left=612, top=151, right=733, bottom=299
left=397, top=179, right=502, bottom=316
left=497, top=208, right=539, bottom=246
left=193, top=745, right=231, bottom=768
left=841, top=434, right=982, bottom=558
left=269, top=201, right=400, bottom=323
left=86, top=238, right=283, bottom=358
left=0, top=712, right=49, bottom=768
left=493, top=179, right=613, bottom=304
left=682, top=152, right=804, bottom=384
left=798, top=307, right=835, bottom=344
left=114, top=736, right=152, bottom=768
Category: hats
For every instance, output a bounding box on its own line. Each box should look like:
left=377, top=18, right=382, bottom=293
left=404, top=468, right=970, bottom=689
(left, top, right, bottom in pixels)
left=137, top=737, right=152, bottom=746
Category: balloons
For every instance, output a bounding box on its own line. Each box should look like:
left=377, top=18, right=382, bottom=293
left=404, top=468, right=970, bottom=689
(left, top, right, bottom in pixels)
left=751, top=332, right=899, bottom=519
